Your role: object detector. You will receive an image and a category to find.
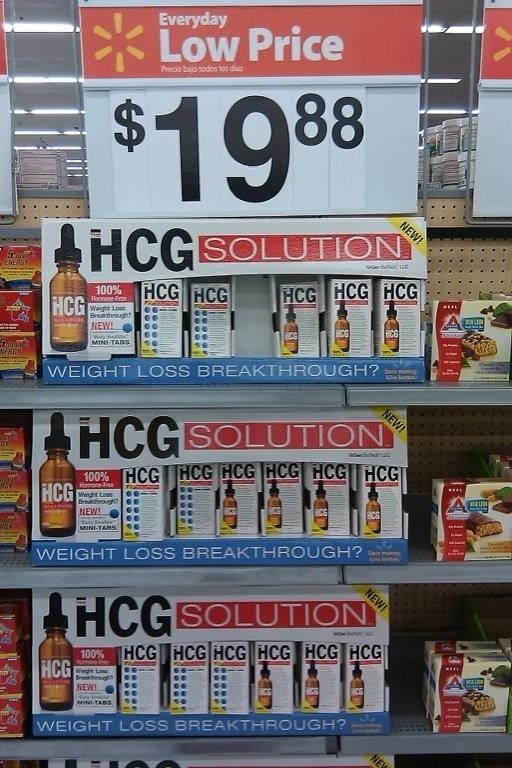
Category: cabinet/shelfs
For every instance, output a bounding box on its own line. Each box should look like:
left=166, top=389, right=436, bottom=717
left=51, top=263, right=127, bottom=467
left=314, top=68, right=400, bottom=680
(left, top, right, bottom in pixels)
left=0, top=190, right=512, bottom=766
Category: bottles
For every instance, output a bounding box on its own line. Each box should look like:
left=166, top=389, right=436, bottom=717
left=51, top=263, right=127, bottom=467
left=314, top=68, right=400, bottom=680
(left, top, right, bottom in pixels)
left=257, top=660, right=273, bottom=709
left=283, top=303, right=299, bottom=354
left=305, top=659, right=321, bottom=709
left=49, top=221, right=89, bottom=351
left=37, top=591, right=74, bottom=710
left=335, top=300, right=350, bottom=352
left=350, top=661, right=365, bottom=708
left=384, top=299, right=400, bottom=353
left=313, top=480, right=329, bottom=531
left=37, top=409, right=77, bottom=537
left=221, top=478, right=237, bottom=528
left=365, top=482, right=382, bottom=532
left=267, top=478, right=282, bottom=528
